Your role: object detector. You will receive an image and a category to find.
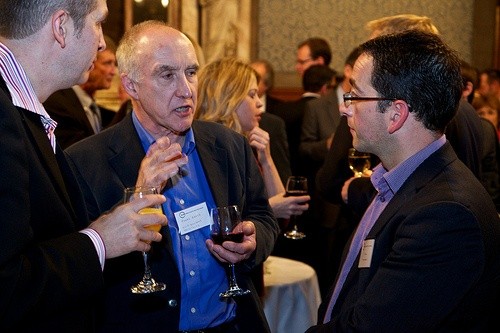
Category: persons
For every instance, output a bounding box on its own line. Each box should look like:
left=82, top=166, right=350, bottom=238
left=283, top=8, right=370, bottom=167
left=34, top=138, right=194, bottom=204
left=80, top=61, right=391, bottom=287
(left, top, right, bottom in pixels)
left=250, top=15, right=500, bottom=282
left=42, top=35, right=131, bottom=168
left=0, top=0, right=167, bottom=333
left=302, top=29, right=500, bottom=333
left=61, top=20, right=279, bottom=333
left=195, top=60, right=311, bottom=220
left=197, top=59, right=310, bottom=220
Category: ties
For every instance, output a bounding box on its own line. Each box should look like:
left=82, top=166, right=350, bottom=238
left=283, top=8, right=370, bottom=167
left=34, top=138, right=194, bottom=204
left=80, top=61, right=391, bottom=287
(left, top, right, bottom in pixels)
left=89, top=104, right=102, bottom=133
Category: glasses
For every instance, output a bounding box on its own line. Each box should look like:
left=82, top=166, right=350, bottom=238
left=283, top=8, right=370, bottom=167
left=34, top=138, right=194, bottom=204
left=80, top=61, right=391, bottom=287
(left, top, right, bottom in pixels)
left=343, top=92, right=397, bottom=108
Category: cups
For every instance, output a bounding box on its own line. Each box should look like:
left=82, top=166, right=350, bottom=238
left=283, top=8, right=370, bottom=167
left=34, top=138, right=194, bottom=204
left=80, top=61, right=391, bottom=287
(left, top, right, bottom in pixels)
left=348, top=147, right=371, bottom=178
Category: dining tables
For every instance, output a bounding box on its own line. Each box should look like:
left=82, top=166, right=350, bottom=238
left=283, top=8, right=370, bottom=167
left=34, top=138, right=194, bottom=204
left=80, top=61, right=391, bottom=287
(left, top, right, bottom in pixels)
left=259, top=255, right=321, bottom=333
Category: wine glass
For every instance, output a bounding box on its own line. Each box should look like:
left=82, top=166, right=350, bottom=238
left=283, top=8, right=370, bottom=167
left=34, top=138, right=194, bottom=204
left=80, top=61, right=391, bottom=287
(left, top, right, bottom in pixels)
left=123, top=186, right=167, bottom=295
left=209, top=205, right=252, bottom=298
left=283, top=175, right=308, bottom=240
